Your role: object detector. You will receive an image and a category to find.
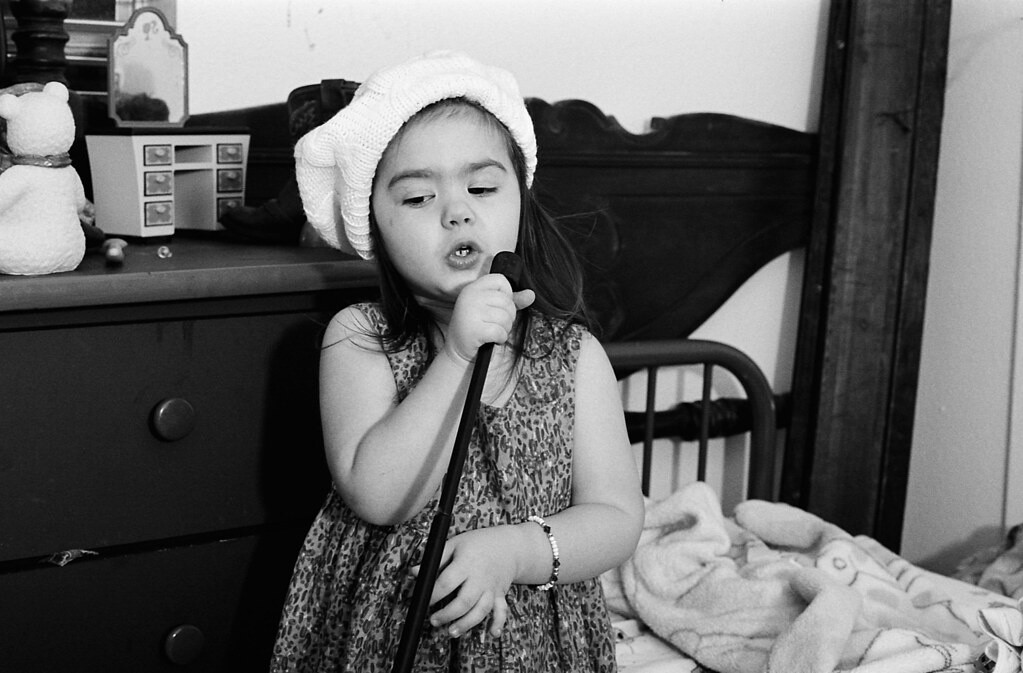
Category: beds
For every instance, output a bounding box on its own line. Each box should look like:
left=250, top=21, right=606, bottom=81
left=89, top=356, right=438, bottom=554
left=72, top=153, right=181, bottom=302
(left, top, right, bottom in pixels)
left=593, top=333, right=1023, bottom=673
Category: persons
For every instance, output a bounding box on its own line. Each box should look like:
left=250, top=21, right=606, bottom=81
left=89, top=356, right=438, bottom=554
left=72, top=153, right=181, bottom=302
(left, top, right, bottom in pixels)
left=270, top=57, right=645, bottom=673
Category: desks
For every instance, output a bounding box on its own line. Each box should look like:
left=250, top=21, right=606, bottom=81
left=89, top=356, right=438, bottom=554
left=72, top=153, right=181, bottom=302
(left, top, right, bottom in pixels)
left=85, top=134, right=253, bottom=236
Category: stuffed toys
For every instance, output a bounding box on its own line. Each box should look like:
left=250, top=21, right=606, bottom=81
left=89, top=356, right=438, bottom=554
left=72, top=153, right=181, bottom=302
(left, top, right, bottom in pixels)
left=0, top=82, right=86, bottom=275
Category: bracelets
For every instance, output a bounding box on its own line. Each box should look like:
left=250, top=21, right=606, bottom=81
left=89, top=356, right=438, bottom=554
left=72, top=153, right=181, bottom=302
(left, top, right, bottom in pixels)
left=523, top=515, right=560, bottom=592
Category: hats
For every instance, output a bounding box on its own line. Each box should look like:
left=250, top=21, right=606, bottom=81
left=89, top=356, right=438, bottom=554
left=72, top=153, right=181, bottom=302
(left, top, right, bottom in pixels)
left=293, top=47, right=538, bottom=259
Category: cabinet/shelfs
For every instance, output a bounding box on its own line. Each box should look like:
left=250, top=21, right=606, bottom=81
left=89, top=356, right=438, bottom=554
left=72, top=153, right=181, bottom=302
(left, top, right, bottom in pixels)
left=0, top=237, right=381, bottom=672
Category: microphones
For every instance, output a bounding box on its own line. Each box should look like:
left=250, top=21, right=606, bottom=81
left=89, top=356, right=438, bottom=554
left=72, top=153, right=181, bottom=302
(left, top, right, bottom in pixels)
left=489, top=250, right=521, bottom=284
